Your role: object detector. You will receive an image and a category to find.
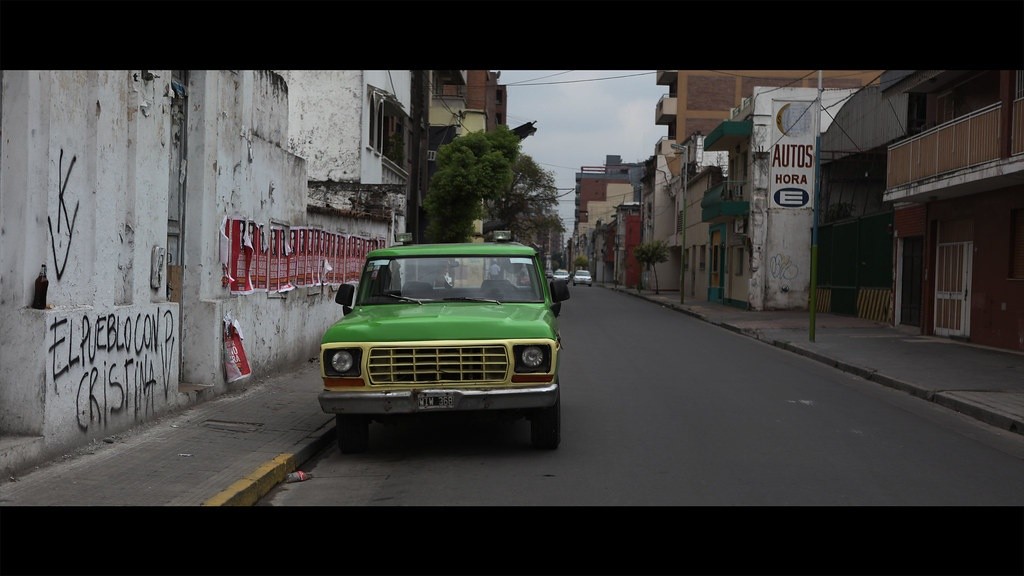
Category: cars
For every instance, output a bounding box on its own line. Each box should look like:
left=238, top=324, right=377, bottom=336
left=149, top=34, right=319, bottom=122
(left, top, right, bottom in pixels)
left=552, top=269, right=570, bottom=284
left=546, top=270, right=553, bottom=278
left=572, top=269, right=592, bottom=287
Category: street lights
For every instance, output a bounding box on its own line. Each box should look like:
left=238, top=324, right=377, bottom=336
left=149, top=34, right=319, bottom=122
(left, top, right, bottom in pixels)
left=670, top=143, right=689, bottom=303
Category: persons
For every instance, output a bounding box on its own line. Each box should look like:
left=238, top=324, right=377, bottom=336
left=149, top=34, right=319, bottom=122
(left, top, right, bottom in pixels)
left=308, top=230, right=312, bottom=254
left=270, top=230, right=276, bottom=255
left=489, top=260, right=500, bottom=281
left=259, top=226, right=266, bottom=252
left=300, top=231, right=305, bottom=253
left=280, top=230, right=286, bottom=255
left=321, top=231, right=385, bottom=258
left=315, top=230, right=319, bottom=254
left=238, top=222, right=245, bottom=250
left=290, top=231, right=296, bottom=255
left=249, top=224, right=255, bottom=251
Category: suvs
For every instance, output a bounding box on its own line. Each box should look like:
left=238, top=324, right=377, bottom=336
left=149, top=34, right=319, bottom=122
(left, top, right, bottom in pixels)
left=317, top=240, right=572, bottom=451
